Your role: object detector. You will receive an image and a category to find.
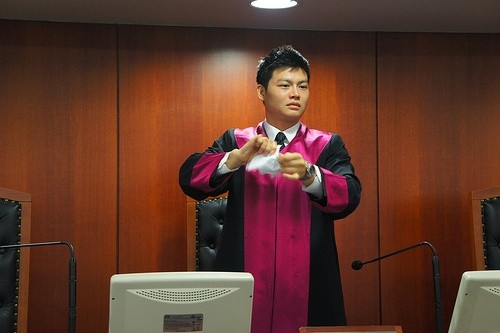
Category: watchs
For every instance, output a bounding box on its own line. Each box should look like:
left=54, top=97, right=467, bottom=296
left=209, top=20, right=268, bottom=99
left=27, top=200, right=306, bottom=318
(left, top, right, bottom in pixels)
left=299, top=163, right=315, bottom=181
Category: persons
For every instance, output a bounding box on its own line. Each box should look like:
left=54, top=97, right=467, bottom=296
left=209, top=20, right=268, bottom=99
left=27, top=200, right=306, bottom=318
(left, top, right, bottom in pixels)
left=179, top=46, right=363, bottom=333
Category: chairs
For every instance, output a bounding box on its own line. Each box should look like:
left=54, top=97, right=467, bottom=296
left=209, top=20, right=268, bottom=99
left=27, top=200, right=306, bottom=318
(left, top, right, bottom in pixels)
left=0, top=187, right=32, bottom=333
left=470, top=184, right=500, bottom=269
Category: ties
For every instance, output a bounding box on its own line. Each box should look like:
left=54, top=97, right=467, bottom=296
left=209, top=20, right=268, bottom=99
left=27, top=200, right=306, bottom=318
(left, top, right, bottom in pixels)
left=274, top=130, right=286, bottom=153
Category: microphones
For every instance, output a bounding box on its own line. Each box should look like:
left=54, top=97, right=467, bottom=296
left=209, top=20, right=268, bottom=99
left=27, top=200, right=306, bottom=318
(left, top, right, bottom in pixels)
left=352, top=241, right=444, bottom=333
left=1, top=240, right=76, bottom=333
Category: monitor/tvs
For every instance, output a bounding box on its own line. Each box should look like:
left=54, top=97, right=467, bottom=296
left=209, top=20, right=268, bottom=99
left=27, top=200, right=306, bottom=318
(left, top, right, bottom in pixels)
left=109, top=272, right=254, bottom=333
left=448, top=270, right=500, bottom=333
